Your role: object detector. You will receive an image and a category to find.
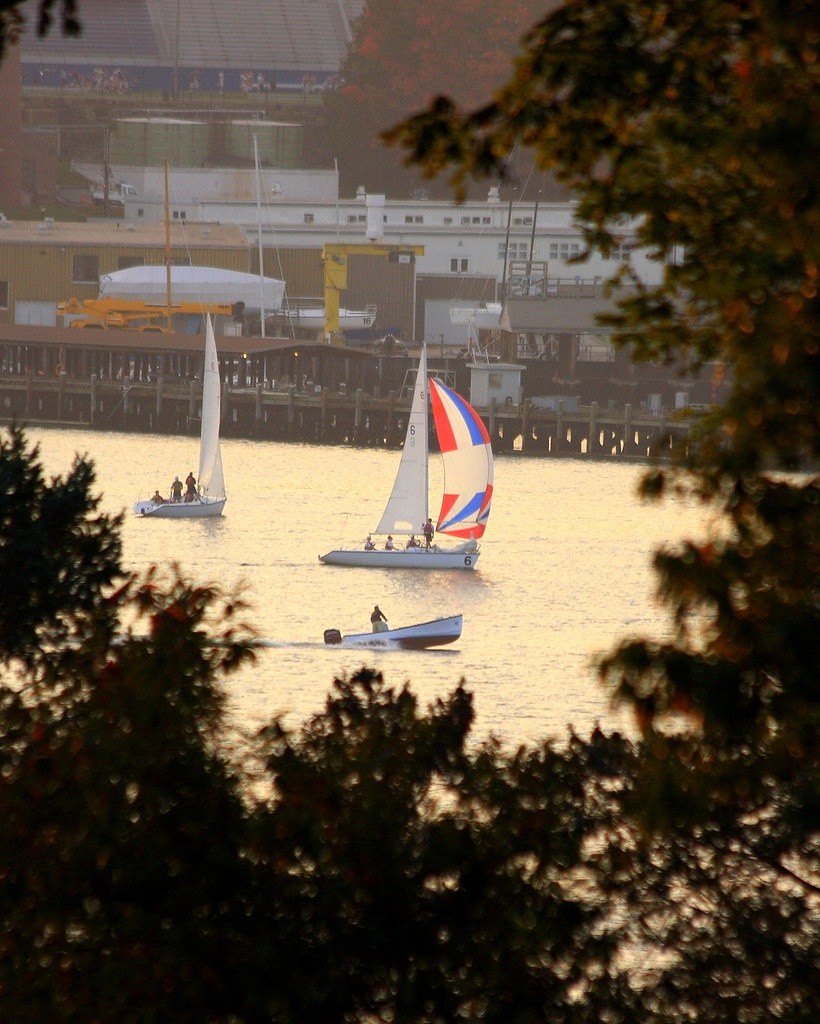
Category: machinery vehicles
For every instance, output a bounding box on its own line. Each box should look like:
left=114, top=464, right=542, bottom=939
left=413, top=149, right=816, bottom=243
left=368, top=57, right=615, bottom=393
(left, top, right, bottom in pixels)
left=312, top=239, right=427, bottom=345
left=54, top=295, right=246, bottom=335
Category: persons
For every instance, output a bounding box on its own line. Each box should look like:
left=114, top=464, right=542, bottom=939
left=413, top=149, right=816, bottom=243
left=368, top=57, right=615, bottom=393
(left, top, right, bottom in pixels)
left=371, top=605, right=388, bottom=624
left=385, top=535, right=399, bottom=550
left=423, top=518, right=434, bottom=548
left=365, top=536, right=376, bottom=550
left=151, top=471, right=197, bottom=504
left=406, top=535, right=417, bottom=550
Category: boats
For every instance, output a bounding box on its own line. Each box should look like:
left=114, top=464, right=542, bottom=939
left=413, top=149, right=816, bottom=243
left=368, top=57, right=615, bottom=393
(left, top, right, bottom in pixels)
left=267, top=293, right=379, bottom=334
left=94, top=265, right=287, bottom=312
left=322, top=613, right=465, bottom=652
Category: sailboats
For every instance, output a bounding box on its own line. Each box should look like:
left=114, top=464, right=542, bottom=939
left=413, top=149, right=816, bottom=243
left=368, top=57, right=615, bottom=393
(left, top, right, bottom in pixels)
left=316, top=340, right=495, bottom=571
left=129, top=312, right=228, bottom=519
left=225, top=134, right=327, bottom=399
left=446, top=134, right=540, bottom=331
